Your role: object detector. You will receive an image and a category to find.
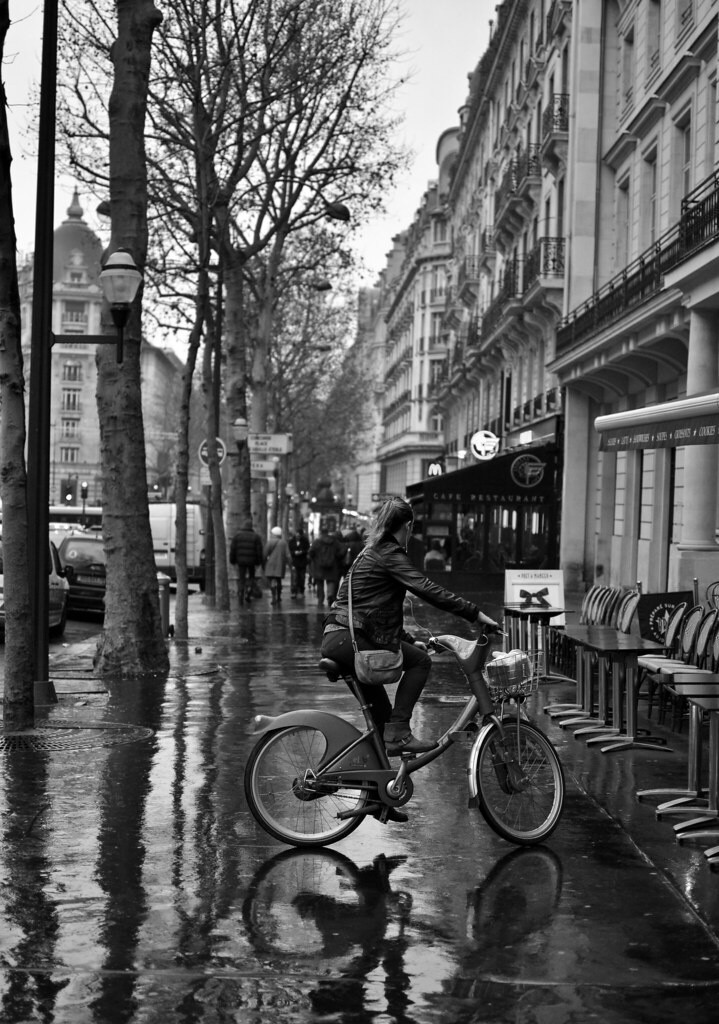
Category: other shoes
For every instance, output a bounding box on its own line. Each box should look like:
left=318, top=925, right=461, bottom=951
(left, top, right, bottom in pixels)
left=290, top=593, right=296, bottom=598
left=298, top=592, right=305, bottom=598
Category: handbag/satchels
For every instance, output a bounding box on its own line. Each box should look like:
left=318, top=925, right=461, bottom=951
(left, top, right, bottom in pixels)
left=261, top=557, right=266, bottom=570
left=340, top=548, right=351, bottom=568
left=353, top=649, right=403, bottom=685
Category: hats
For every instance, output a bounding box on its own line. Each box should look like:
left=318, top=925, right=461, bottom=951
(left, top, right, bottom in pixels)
left=242, top=518, right=252, bottom=528
left=271, top=525, right=282, bottom=536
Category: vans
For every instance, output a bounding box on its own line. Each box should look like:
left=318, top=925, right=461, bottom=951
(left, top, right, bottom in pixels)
left=0, top=530, right=72, bottom=634
left=148, top=501, right=204, bottom=592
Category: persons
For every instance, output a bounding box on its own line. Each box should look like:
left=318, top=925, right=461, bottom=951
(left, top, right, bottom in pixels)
left=311, top=526, right=365, bottom=607
left=229, top=520, right=263, bottom=607
left=289, top=529, right=310, bottom=599
left=263, top=526, right=293, bottom=605
left=423, top=540, right=472, bottom=573
left=321, top=497, right=498, bottom=822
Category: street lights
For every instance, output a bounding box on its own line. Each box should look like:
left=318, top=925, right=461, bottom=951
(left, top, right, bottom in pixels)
left=202, top=178, right=354, bottom=610
left=31, top=244, right=139, bottom=708
left=283, top=480, right=294, bottom=534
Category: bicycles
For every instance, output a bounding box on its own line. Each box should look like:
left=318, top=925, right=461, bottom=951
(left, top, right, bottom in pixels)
left=241, top=622, right=567, bottom=849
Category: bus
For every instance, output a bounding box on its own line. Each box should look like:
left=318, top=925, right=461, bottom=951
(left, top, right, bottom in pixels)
left=48, top=504, right=104, bottom=543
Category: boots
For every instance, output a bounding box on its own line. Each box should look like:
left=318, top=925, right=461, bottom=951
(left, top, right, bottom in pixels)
left=245, top=584, right=252, bottom=603
left=365, top=792, right=409, bottom=822
left=383, top=721, right=440, bottom=757
left=277, top=586, right=281, bottom=601
left=237, top=587, right=244, bottom=605
left=270, top=586, right=276, bottom=606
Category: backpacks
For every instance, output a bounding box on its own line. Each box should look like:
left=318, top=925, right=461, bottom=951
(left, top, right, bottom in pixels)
left=319, top=539, right=339, bottom=569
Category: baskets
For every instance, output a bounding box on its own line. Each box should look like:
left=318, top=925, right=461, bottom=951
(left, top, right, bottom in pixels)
left=482, top=648, right=544, bottom=701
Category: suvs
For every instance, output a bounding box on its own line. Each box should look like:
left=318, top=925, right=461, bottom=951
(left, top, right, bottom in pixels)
left=55, top=534, right=113, bottom=618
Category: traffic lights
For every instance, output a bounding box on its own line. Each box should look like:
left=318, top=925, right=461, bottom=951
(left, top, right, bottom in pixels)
left=59, top=470, right=77, bottom=508
left=78, top=478, right=92, bottom=507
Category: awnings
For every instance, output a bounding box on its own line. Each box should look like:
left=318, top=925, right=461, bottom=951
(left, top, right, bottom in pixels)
left=405, top=443, right=560, bottom=508
left=593, top=387, right=719, bottom=452
